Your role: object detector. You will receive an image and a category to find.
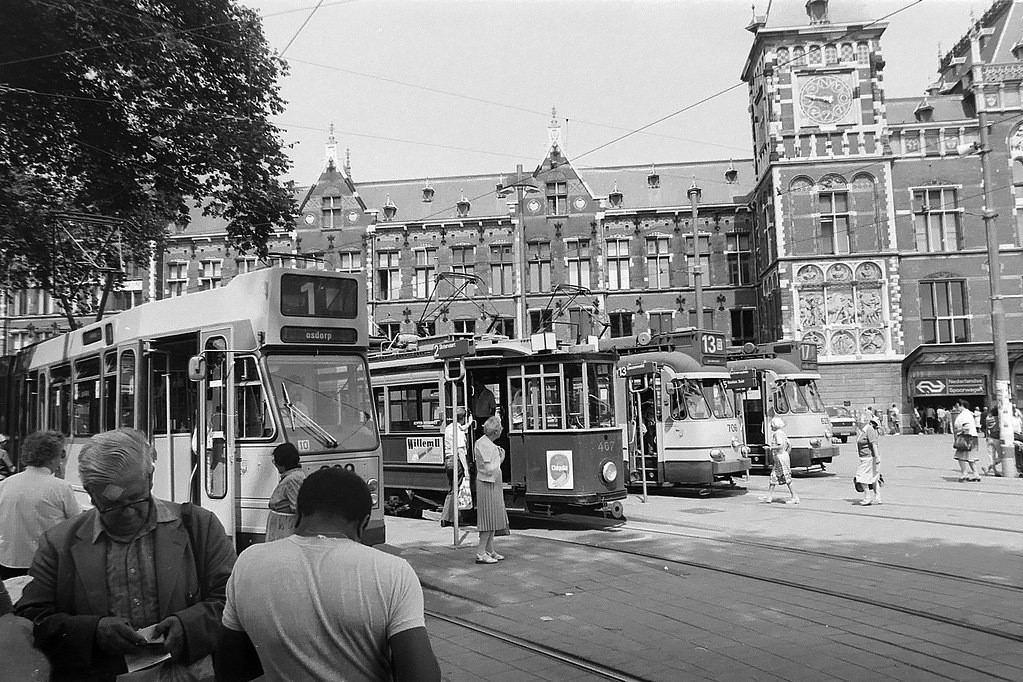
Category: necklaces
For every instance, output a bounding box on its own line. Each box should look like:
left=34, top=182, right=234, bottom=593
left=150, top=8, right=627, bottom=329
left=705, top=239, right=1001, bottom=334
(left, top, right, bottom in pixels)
left=302, top=529, right=348, bottom=538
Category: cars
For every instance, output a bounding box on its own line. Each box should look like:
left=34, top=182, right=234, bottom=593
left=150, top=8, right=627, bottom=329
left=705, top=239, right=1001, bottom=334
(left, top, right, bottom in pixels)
left=825, top=406, right=857, bottom=444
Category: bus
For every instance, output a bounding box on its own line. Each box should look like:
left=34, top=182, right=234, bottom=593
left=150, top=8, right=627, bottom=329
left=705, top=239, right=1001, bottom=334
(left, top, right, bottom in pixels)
left=0, top=209, right=388, bottom=556
left=317, top=270, right=629, bottom=521
left=421, top=282, right=840, bottom=496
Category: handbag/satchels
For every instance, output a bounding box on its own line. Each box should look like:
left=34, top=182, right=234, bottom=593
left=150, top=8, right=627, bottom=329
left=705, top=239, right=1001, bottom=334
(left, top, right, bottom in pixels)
left=181, top=502, right=264, bottom=682
left=952, top=428, right=973, bottom=451
left=854, top=472, right=884, bottom=492
left=116, top=654, right=215, bottom=682
left=458, top=477, right=473, bottom=510
left=479, top=511, right=510, bottom=537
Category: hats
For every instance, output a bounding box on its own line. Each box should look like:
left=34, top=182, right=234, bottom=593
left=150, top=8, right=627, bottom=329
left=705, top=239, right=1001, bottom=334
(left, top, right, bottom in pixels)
left=0, top=434, right=10, bottom=443
left=456, top=407, right=465, bottom=422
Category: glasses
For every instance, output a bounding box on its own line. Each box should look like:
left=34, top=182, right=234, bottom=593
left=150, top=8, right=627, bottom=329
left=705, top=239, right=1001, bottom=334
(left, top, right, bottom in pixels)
left=85, top=474, right=152, bottom=515
left=271, top=459, right=275, bottom=464
left=53, top=450, right=66, bottom=458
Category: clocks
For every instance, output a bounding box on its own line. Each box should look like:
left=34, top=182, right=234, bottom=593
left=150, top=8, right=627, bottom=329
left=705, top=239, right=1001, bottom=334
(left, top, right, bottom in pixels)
left=799, top=76, right=854, bottom=124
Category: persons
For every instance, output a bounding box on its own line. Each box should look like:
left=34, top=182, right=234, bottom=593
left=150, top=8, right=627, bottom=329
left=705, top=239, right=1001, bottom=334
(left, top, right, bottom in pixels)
left=778, top=385, right=807, bottom=412
left=28, top=427, right=239, bottom=682
left=0, top=429, right=81, bottom=616
left=265, top=443, right=306, bottom=542
left=865, top=399, right=1023, bottom=483
left=527, top=380, right=558, bottom=417
left=853, top=415, right=884, bottom=506
left=757, top=418, right=799, bottom=505
left=276, top=374, right=310, bottom=427
left=473, top=376, right=497, bottom=441
left=221, top=468, right=441, bottom=682
left=634, top=385, right=658, bottom=455
left=440, top=407, right=474, bottom=527
left=475, top=416, right=512, bottom=564
left=188, top=402, right=198, bottom=503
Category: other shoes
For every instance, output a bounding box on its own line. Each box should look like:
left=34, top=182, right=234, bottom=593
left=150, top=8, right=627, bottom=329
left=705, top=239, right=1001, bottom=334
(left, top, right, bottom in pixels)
left=759, top=496, right=772, bottom=503
left=441, top=519, right=450, bottom=527
left=786, top=496, right=800, bottom=505
left=860, top=494, right=882, bottom=505
left=452, top=519, right=470, bottom=526
left=957, top=466, right=1001, bottom=481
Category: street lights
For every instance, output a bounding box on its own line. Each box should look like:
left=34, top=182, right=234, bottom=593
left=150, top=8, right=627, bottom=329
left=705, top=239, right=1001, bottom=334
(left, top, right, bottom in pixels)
left=501, top=164, right=541, bottom=338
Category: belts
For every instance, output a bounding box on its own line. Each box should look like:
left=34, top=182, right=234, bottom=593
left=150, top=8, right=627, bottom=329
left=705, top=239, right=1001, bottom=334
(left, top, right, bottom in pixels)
left=269, top=509, right=296, bottom=516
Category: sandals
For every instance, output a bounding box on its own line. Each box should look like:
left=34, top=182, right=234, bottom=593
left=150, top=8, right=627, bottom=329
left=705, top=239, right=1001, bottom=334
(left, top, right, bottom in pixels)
left=485, top=550, right=504, bottom=559
left=476, top=553, right=498, bottom=564
